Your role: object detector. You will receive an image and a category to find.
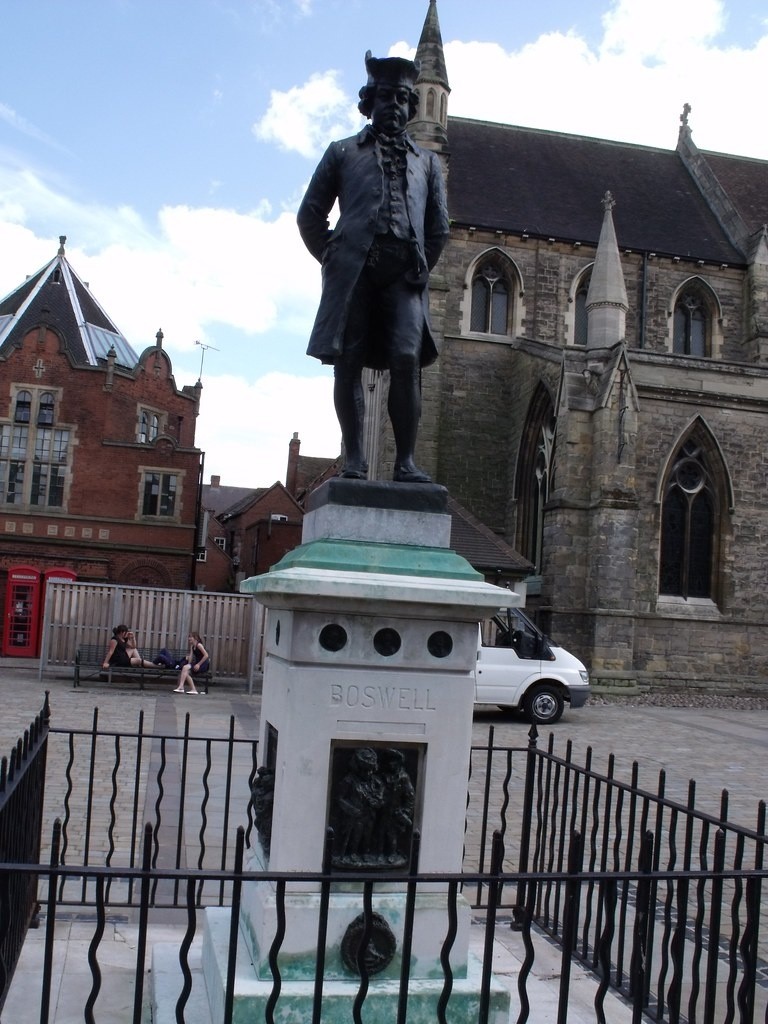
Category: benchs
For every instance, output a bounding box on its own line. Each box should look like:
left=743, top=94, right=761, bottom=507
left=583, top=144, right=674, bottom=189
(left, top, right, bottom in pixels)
left=74, top=644, right=213, bottom=695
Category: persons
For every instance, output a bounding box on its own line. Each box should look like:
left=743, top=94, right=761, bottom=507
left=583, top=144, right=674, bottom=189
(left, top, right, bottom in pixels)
left=173, top=632, right=210, bottom=694
left=298, top=49, right=450, bottom=485
left=102, top=625, right=165, bottom=670
left=332, top=748, right=415, bottom=865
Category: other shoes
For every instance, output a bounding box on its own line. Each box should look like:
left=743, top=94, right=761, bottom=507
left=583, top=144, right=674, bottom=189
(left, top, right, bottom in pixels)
left=186, top=691, right=198, bottom=695
left=173, top=689, right=185, bottom=693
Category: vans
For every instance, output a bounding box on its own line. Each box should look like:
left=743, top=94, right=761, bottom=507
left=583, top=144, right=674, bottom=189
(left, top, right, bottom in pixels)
left=473, top=607, right=591, bottom=726
